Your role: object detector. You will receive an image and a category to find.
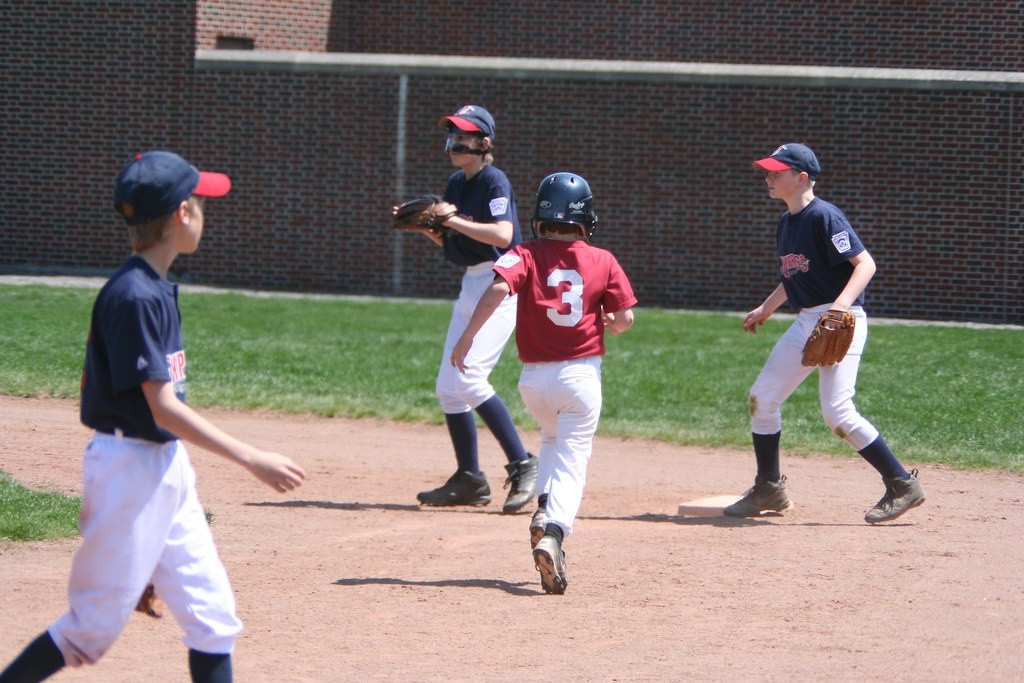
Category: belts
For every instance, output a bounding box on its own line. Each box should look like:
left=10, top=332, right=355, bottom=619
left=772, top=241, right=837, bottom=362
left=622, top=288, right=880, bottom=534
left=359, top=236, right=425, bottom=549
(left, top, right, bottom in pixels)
left=98, top=428, right=144, bottom=439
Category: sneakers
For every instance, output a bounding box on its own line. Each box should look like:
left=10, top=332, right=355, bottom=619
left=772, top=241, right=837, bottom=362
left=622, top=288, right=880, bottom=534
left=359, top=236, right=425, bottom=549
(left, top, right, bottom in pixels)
left=528, top=507, right=546, bottom=549
left=502, top=452, right=541, bottom=513
left=723, top=473, right=792, bottom=518
left=532, top=535, right=568, bottom=595
left=416, top=468, right=491, bottom=506
left=864, top=467, right=927, bottom=524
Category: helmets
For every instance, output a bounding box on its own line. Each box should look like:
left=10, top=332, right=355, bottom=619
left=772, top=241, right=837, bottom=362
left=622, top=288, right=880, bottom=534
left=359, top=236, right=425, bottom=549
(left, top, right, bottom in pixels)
left=533, top=172, right=598, bottom=242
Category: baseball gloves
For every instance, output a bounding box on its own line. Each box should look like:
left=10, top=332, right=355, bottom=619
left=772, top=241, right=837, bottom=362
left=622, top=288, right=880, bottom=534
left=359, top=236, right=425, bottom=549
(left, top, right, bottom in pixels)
left=392, top=195, right=457, bottom=230
left=801, top=309, right=855, bottom=367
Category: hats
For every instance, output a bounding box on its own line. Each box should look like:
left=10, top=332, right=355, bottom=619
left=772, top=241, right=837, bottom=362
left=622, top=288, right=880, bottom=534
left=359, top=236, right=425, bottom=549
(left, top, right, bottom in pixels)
left=438, top=104, right=495, bottom=142
left=753, top=143, right=821, bottom=180
left=114, top=151, right=231, bottom=225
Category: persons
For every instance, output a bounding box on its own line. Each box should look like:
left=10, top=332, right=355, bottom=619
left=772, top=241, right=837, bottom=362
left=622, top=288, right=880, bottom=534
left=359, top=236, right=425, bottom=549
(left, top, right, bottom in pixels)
left=723, top=141, right=926, bottom=524
left=391, top=104, right=542, bottom=515
left=449, top=170, right=638, bottom=596
left=0, top=150, right=306, bottom=683
